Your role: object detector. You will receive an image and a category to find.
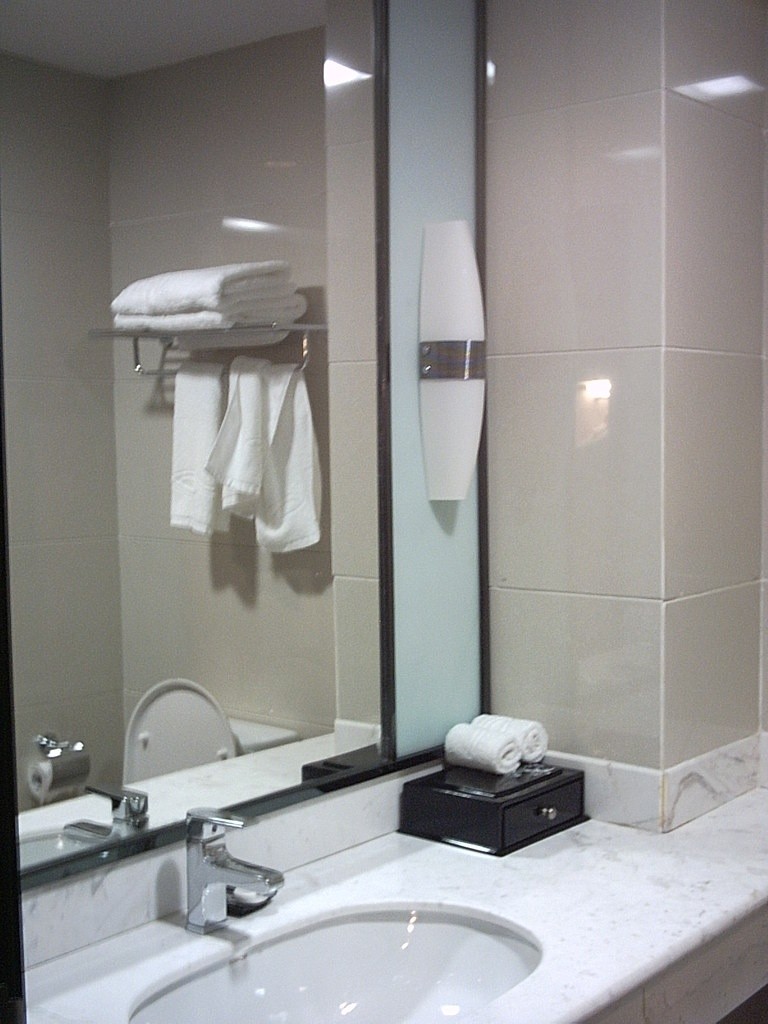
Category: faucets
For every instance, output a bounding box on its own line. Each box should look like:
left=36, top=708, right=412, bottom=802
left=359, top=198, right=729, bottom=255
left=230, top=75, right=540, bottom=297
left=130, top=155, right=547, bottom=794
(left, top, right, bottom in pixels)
left=186, top=809, right=285, bottom=932
left=63, top=784, right=148, bottom=844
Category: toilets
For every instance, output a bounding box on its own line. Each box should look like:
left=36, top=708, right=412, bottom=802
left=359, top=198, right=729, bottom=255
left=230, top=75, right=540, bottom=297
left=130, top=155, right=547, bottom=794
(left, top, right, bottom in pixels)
left=122, top=678, right=297, bottom=788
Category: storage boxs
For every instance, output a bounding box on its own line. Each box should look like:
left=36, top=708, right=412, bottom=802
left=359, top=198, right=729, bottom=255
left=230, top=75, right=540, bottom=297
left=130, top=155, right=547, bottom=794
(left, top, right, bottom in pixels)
left=396, top=764, right=593, bottom=858
left=301, top=743, right=378, bottom=782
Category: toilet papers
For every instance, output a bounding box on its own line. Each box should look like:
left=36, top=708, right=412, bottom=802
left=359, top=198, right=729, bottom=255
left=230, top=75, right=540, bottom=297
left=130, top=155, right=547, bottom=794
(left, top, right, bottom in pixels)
left=25, top=752, right=67, bottom=799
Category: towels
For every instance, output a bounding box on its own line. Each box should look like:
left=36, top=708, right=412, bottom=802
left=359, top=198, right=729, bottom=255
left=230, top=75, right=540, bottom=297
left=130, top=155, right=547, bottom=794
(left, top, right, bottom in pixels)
left=170, top=362, right=232, bottom=537
left=111, top=261, right=308, bottom=349
left=206, top=357, right=323, bottom=554
left=444, top=711, right=551, bottom=773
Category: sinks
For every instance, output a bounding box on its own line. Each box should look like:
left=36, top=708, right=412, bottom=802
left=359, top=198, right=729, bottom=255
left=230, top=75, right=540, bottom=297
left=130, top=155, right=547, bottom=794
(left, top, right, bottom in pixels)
left=19, top=823, right=91, bottom=869
left=127, top=896, right=541, bottom=1024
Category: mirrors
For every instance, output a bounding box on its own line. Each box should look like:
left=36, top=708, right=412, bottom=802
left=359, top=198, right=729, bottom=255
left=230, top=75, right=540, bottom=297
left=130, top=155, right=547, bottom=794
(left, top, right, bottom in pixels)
left=0, top=0, right=397, bottom=971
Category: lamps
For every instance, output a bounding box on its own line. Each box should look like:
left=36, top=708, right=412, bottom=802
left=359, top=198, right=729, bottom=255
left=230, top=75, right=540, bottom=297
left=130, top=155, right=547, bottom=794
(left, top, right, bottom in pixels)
left=416, top=218, right=488, bottom=503
